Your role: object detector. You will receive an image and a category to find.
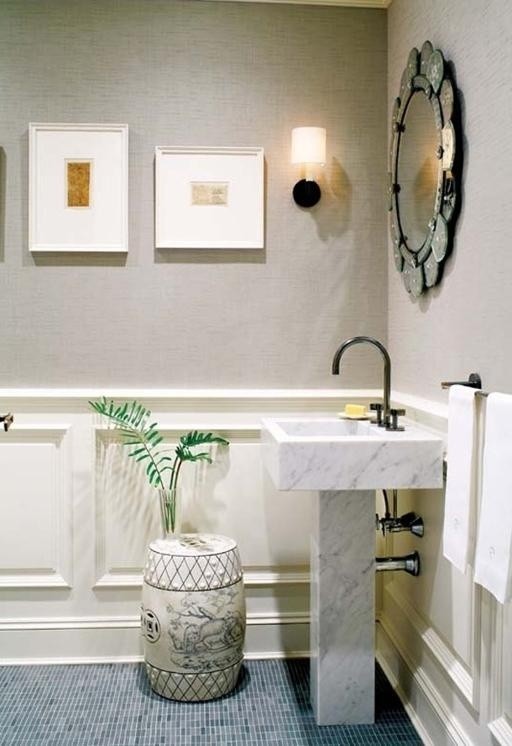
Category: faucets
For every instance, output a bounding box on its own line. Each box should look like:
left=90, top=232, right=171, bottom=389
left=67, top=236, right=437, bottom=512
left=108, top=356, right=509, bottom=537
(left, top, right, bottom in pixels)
left=331, top=336, right=390, bottom=429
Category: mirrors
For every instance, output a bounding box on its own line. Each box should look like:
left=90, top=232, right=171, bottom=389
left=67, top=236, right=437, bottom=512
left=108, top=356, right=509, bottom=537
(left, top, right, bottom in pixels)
left=386, top=40, right=459, bottom=298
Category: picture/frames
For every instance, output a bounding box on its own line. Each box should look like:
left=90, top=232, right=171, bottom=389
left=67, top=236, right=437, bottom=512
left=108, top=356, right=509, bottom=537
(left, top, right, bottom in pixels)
left=26, top=124, right=265, bottom=256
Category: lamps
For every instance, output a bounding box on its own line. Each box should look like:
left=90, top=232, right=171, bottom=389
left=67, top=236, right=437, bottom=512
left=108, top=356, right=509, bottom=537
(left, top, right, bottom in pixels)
left=291, top=126, right=327, bottom=207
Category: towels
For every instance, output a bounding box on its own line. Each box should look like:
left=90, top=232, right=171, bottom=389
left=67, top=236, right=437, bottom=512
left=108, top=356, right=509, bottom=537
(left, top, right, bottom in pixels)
left=433, top=383, right=512, bottom=605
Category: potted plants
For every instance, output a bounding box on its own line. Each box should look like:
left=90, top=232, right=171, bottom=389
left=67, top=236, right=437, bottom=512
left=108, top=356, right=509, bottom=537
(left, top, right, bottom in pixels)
left=88, top=397, right=228, bottom=536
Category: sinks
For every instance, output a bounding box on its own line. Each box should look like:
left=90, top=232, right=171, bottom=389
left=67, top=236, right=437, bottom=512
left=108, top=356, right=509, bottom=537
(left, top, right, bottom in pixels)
left=260, top=421, right=444, bottom=492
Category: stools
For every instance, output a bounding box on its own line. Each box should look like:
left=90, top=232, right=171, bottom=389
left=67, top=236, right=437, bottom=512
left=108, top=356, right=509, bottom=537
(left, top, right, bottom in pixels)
left=143, top=537, right=243, bottom=703
